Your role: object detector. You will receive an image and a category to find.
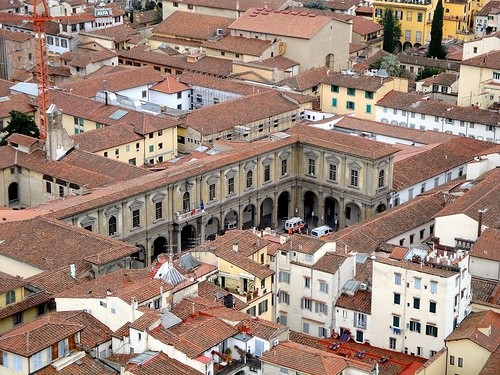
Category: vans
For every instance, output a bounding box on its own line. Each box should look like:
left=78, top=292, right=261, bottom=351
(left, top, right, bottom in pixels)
left=285, top=217, right=306, bottom=232
left=312, top=226, right=333, bottom=237
left=222, top=221, right=244, bottom=230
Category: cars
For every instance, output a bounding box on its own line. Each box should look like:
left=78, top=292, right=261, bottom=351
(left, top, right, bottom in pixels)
left=331, top=342, right=340, bottom=350
left=380, top=355, right=390, bottom=364
left=356, top=350, right=366, bottom=359
